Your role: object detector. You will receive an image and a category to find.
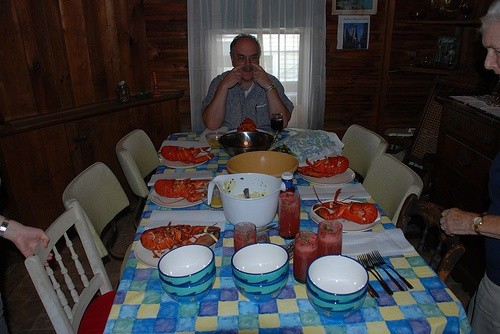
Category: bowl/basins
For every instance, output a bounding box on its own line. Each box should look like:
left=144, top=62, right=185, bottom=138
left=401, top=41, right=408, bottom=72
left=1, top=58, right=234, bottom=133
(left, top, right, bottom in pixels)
left=206, top=132, right=226, bottom=148
left=158, top=245, right=217, bottom=303
left=219, top=132, right=274, bottom=158
left=226, top=151, right=300, bottom=179
left=306, top=254, right=369, bottom=321
left=230, top=242, right=289, bottom=303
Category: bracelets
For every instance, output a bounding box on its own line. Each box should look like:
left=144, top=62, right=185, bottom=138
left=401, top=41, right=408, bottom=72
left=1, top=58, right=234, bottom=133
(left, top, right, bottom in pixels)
left=0, top=217, right=10, bottom=237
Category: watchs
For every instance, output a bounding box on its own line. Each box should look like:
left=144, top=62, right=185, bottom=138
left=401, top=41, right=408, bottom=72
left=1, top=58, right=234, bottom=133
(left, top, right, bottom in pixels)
left=265, top=83, right=276, bottom=91
left=473, top=213, right=487, bottom=235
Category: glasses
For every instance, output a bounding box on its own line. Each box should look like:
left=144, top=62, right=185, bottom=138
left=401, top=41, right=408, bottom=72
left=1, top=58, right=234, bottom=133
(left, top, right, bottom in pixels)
left=231, top=54, right=259, bottom=63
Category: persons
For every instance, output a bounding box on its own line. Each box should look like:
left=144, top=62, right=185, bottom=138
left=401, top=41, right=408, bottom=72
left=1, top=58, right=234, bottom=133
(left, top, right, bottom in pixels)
left=0, top=215, right=53, bottom=333
left=202, top=34, right=294, bottom=131
left=440, top=0, right=500, bottom=334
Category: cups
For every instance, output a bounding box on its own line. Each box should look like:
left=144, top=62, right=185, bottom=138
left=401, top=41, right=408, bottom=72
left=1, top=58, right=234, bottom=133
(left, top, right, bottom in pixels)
left=234, top=222, right=257, bottom=251
left=294, top=231, right=319, bottom=284
left=210, top=185, right=223, bottom=208
left=317, top=220, right=342, bottom=258
left=279, top=190, right=301, bottom=240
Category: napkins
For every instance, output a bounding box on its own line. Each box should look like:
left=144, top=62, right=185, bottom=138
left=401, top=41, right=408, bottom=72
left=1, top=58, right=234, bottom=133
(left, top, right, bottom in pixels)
left=159, top=140, right=211, bottom=152
left=150, top=208, right=224, bottom=231
left=341, top=228, right=418, bottom=256
left=296, top=181, right=372, bottom=202
left=147, top=169, right=212, bottom=186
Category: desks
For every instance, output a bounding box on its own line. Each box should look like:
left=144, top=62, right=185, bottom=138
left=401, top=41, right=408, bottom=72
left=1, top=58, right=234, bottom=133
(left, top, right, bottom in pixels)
left=100, top=128, right=471, bottom=334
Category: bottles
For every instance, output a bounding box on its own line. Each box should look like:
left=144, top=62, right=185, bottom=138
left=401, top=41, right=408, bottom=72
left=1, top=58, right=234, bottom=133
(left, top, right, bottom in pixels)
left=278, top=173, right=298, bottom=193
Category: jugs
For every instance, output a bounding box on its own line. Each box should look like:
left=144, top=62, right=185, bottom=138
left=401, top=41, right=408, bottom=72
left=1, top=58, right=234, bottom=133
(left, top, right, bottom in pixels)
left=208, top=173, right=286, bottom=228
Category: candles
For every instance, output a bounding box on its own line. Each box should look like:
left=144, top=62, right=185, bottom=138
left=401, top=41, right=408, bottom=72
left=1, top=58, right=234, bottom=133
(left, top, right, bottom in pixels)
left=153, top=71, right=157, bottom=85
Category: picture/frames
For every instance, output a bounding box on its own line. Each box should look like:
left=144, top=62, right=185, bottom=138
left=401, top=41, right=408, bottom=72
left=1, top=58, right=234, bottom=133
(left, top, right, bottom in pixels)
left=332, top=0, right=378, bottom=15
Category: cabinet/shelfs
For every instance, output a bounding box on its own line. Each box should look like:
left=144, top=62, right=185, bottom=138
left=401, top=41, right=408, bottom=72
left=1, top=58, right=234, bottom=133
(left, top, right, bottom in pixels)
left=431, top=93, right=500, bottom=213
left=398, top=16, right=482, bottom=76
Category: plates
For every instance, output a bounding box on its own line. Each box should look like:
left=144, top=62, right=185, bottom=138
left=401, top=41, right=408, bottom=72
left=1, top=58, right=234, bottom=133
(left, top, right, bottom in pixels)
left=149, top=188, right=208, bottom=208
left=298, top=167, right=356, bottom=185
left=228, top=128, right=268, bottom=134
left=160, top=151, right=208, bottom=168
left=310, top=199, right=381, bottom=232
left=134, top=240, right=167, bottom=269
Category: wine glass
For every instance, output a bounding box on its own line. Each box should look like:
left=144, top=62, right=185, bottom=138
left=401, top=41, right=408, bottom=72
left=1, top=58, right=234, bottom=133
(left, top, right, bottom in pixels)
left=270, top=113, right=283, bottom=147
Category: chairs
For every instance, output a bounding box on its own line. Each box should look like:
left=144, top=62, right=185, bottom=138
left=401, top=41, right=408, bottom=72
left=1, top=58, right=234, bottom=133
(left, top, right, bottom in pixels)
left=396, top=194, right=467, bottom=282
left=63, top=161, right=137, bottom=284
left=341, top=125, right=388, bottom=180
left=24, top=201, right=117, bottom=334
left=115, top=128, right=160, bottom=230
left=401, top=72, right=476, bottom=169
left=361, top=154, right=423, bottom=229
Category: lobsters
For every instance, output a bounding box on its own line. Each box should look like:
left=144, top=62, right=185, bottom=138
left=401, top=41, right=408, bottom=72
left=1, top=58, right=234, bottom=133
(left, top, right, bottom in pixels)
left=297, top=155, right=349, bottom=177
left=139, top=221, right=221, bottom=258
left=152, top=178, right=212, bottom=204
left=312, top=185, right=378, bottom=225
left=237, top=118, right=256, bottom=132
left=161, top=145, right=214, bottom=164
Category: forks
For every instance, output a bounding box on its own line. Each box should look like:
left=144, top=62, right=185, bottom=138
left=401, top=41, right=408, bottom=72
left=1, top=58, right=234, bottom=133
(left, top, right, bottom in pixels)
left=356, top=250, right=413, bottom=295
